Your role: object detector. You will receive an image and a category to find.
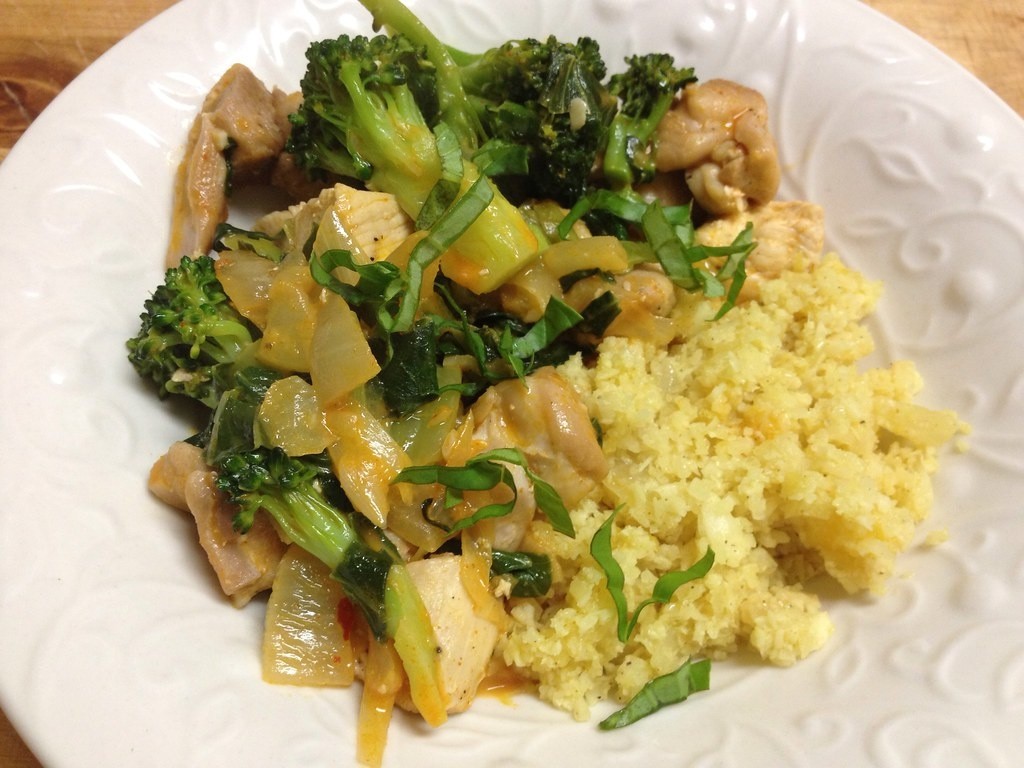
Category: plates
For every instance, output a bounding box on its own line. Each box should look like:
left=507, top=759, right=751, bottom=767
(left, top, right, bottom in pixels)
left=0, top=0, right=1024, bottom=768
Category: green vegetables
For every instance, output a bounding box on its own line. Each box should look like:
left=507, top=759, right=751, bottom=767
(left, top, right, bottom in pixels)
left=210, top=125, right=763, bottom=724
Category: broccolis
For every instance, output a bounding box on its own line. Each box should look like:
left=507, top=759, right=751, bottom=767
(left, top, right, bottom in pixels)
left=126, top=0, right=702, bottom=637
